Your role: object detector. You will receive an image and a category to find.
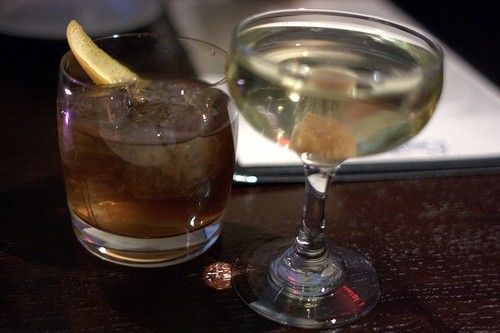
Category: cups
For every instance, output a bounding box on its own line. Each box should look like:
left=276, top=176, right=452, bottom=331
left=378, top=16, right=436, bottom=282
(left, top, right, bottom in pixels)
left=59, top=30, right=240, bottom=270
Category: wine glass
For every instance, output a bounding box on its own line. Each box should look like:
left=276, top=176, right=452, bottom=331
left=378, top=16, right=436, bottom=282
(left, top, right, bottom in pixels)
left=222, top=10, right=446, bottom=331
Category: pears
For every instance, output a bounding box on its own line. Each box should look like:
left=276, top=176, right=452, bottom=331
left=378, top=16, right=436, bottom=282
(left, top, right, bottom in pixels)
left=64, top=18, right=141, bottom=85
